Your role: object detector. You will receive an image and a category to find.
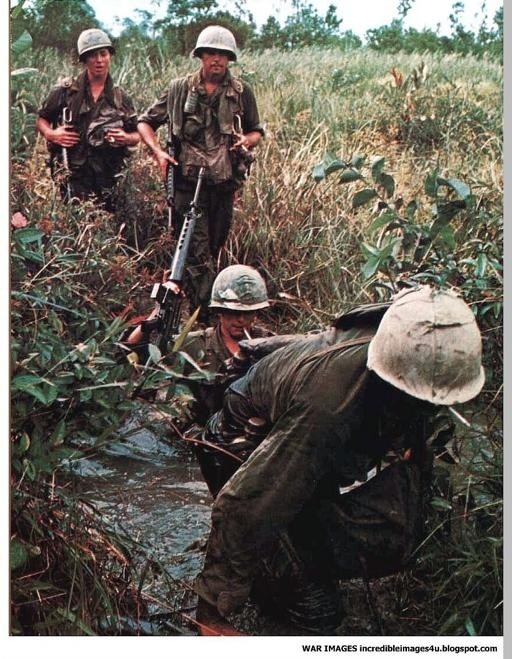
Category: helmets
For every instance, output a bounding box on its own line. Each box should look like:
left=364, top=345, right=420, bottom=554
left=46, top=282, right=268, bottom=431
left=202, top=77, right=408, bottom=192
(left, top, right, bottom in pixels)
left=368, top=287, right=485, bottom=405
left=208, top=264, right=270, bottom=311
left=77, top=29, right=114, bottom=59
left=192, top=25, right=237, bottom=60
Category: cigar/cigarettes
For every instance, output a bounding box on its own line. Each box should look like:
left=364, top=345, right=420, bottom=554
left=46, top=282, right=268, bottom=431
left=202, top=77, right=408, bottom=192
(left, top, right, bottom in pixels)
left=242, top=326, right=252, bottom=340
left=241, top=144, right=249, bottom=153
left=110, top=136, right=116, bottom=143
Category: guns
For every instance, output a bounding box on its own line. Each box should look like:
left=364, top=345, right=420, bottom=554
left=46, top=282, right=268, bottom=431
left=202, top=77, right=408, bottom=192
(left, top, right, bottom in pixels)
left=165, top=119, right=177, bottom=230
left=60, top=107, right=73, bottom=204
left=115, top=166, right=205, bottom=402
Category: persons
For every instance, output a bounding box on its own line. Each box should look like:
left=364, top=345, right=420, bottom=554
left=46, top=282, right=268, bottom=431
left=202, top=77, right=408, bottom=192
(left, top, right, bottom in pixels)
left=35, top=27, right=142, bottom=218
left=117, top=264, right=277, bottom=423
left=136, top=24, right=266, bottom=259
left=187, top=282, right=488, bottom=636
